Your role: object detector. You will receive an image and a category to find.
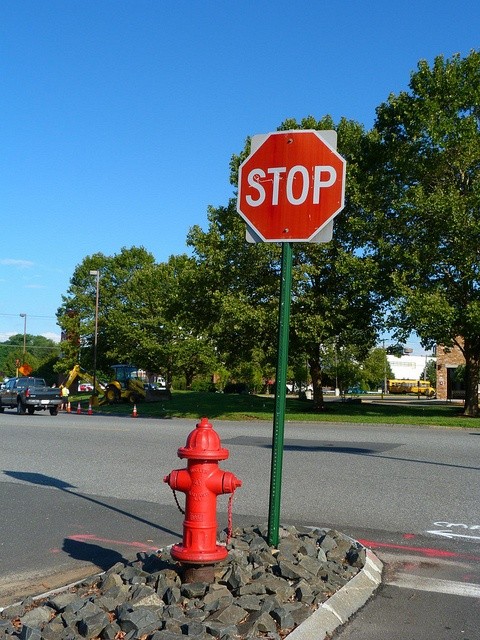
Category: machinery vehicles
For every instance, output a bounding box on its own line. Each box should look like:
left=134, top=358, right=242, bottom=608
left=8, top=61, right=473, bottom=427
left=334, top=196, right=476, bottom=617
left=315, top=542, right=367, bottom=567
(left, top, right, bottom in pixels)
left=64, top=364, right=173, bottom=405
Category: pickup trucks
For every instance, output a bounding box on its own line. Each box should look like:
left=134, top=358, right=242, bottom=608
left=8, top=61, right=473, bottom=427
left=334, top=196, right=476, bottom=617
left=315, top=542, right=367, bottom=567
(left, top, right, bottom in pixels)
left=0, top=376, right=62, bottom=415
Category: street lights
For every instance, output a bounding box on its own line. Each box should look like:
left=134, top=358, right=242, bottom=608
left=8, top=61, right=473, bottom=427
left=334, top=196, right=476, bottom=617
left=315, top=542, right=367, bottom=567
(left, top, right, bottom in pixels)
left=20, top=314, right=26, bottom=376
left=89, top=271, right=99, bottom=407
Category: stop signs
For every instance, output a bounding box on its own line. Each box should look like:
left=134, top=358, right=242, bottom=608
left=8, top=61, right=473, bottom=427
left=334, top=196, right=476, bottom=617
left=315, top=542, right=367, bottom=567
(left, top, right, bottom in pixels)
left=237, top=131, right=348, bottom=243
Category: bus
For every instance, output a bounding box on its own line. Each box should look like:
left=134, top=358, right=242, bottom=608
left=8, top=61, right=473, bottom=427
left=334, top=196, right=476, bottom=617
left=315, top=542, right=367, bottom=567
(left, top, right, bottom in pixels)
left=388, top=379, right=434, bottom=395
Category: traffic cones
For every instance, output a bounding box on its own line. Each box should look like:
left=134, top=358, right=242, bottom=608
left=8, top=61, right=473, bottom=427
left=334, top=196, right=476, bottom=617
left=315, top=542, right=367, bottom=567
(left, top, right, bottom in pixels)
left=87, top=403, right=92, bottom=415
left=67, top=402, right=71, bottom=414
left=132, top=404, right=137, bottom=417
left=77, top=402, right=82, bottom=414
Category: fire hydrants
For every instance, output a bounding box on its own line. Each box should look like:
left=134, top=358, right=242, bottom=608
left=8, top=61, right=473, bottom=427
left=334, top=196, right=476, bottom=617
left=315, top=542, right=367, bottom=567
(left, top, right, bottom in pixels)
left=161, top=417, right=241, bottom=564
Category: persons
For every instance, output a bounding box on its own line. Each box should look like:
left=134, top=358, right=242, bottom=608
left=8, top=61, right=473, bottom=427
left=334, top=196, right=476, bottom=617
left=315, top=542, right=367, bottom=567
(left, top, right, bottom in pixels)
left=60, top=384, right=69, bottom=410
left=51, top=384, right=56, bottom=388
